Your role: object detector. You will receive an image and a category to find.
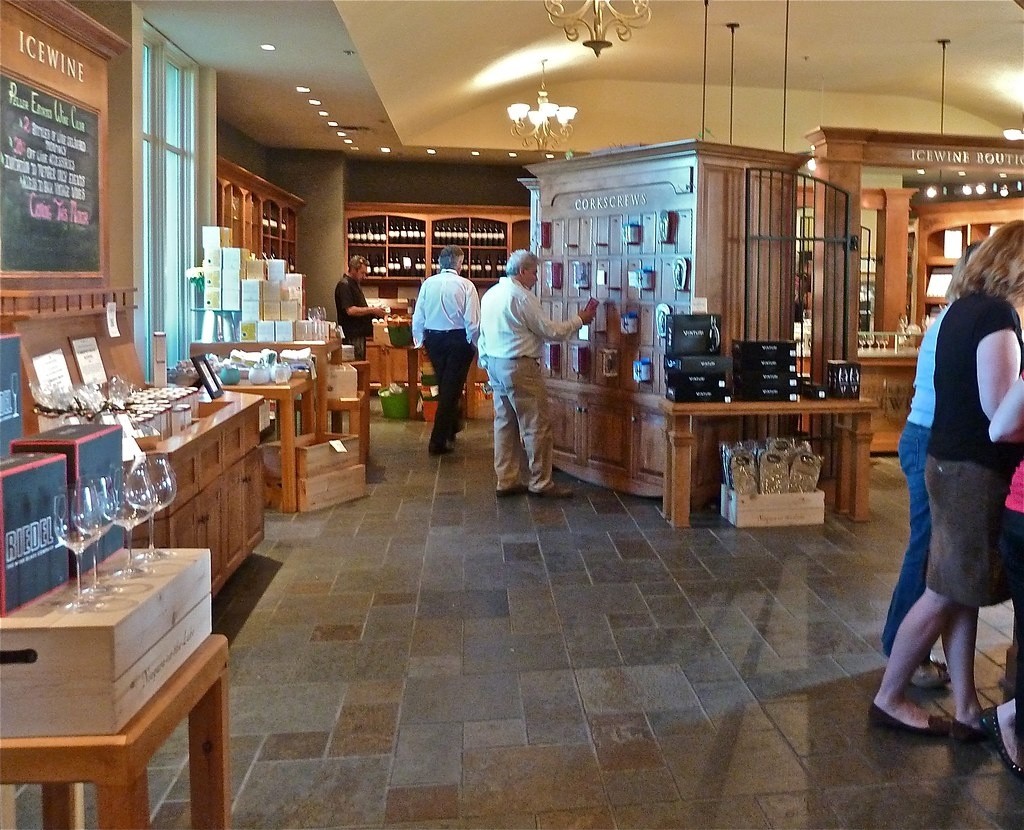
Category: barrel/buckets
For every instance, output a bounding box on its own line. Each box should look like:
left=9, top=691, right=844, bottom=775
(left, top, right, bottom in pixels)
left=377, top=385, right=409, bottom=419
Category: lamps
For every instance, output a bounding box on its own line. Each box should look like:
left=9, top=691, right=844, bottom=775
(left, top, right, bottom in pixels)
left=543, top=0, right=652, bottom=58
left=506, top=58, right=579, bottom=152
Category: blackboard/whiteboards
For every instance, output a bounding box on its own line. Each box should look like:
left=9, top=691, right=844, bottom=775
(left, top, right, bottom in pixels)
left=0, top=66, right=104, bottom=279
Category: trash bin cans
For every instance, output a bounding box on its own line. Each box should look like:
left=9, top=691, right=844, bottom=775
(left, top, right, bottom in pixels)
left=378, top=387, right=410, bottom=419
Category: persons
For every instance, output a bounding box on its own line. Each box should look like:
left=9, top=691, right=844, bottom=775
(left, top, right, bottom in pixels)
left=335, top=255, right=386, bottom=362
left=477, top=249, right=596, bottom=497
left=866, top=219, right=1024, bottom=775
left=411, top=244, right=481, bottom=455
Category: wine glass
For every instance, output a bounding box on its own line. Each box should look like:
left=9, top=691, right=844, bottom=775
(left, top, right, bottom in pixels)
left=69, top=473, right=119, bottom=599
left=308, top=305, right=328, bottom=342
left=53, top=484, right=105, bottom=611
left=102, top=460, right=155, bottom=580
left=123, top=452, right=179, bottom=563
left=859, top=334, right=888, bottom=352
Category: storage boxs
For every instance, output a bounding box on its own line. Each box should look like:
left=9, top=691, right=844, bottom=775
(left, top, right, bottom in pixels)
left=324, top=362, right=358, bottom=400
left=720, top=484, right=826, bottom=529
left=256, top=431, right=368, bottom=514
left=664, top=316, right=861, bottom=403
left=201, top=225, right=336, bottom=345
left=1, top=333, right=212, bottom=739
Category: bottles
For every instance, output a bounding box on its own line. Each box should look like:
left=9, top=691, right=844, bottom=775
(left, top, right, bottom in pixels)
left=349, top=253, right=386, bottom=276
left=388, top=250, right=425, bottom=277
left=431, top=253, right=468, bottom=277
left=348, top=220, right=386, bottom=244
left=127, top=386, right=199, bottom=443
left=389, top=220, right=426, bottom=245
left=433, top=221, right=469, bottom=245
left=263, top=211, right=295, bottom=273
left=471, top=253, right=507, bottom=278
left=470, top=223, right=505, bottom=246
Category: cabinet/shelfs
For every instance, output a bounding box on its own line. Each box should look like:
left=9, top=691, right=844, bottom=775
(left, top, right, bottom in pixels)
left=523, top=139, right=813, bottom=511
left=130, top=391, right=265, bottom=599
left=216, top=155, right=305, bottom=274
left=342, top=201, right=530, bottom=382
left=914, top=196, right=1024, bottom=332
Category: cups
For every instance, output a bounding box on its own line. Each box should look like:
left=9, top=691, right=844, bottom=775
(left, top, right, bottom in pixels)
left=803, top=309, right=812, bottom=324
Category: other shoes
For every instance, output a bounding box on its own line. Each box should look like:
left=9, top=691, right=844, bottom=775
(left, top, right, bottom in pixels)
left=431, top=445, right=454, bottom=456
left=447, top=432, right=457, bottom=441
left=496, top=483, right=529, bottom=498
left=950, top=716, right=992, bottom=742
left=529, top=486, right=573, bottom=498
left=868, top=700, right=951, bottom=737
left=881, top=653, right=949, bottom=689
left=980, top=704, right=1024, bottom=778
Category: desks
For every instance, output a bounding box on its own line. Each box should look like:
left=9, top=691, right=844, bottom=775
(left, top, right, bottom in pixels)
left=218, top=378, right=313, bottom=515
left=657, top=399, right=878, bottom=529
left=190, top=334, right=343, bottom=440
left=366, top=342, right=424, bottom=420
left=275, top=390, right=368, bottom=464
left=0, top=633, right=229, bottom=830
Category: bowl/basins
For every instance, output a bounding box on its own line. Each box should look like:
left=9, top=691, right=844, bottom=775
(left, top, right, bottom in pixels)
left=220, top=358, right=293, bottom=385
